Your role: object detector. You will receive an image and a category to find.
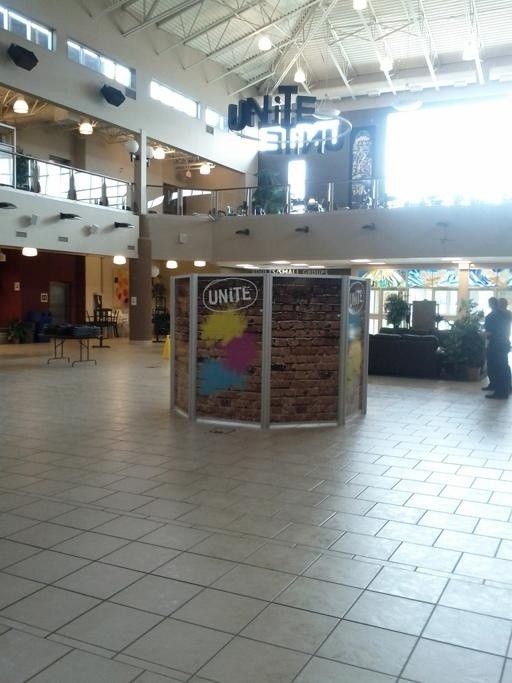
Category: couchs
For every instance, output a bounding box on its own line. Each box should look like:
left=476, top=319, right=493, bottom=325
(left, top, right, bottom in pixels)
left=368, top=328, right=448, bottom=378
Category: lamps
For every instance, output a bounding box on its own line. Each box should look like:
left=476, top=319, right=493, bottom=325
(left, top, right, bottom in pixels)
left=127, top=141, right=139, bottom=162
left=154, top=143, right=165, bottom=159
left=146, top=146, right=154, bottom=167
left=0, top=202, right=16, bottom=209
left=79, top=116, right=93, bottom=135
left=200, top=161, right=210, bottom=175
left=115, top=222, right=134, bottom=229
left=60, top=213, right=82, bottom=221
left=13, top=95, right=28, bottom=114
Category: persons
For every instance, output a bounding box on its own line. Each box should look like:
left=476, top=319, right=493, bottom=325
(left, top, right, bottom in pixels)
left=480, top=295, right=511, bottom=391
left=483, top=297, right=511, bottom=401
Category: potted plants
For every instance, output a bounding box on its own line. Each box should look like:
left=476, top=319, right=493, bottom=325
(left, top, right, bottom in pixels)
left=434, top=298, right=484, bottom=382
left=7, top=319, right=31, bottom=344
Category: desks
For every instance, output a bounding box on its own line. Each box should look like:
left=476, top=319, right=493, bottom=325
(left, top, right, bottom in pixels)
left=46, top=332, right=103, bottom=367
